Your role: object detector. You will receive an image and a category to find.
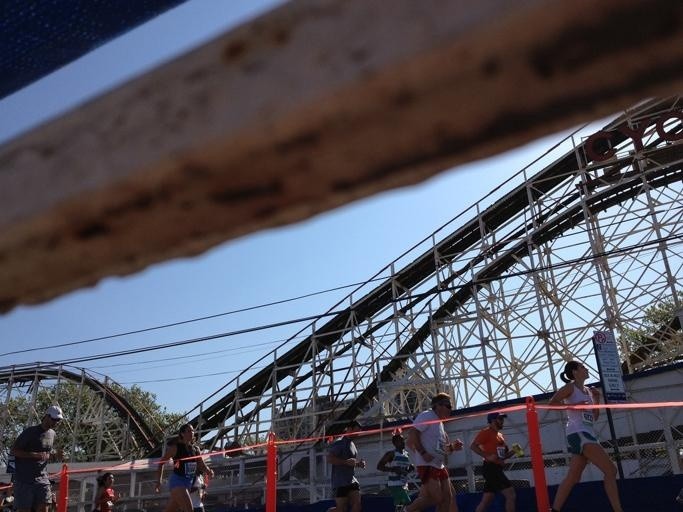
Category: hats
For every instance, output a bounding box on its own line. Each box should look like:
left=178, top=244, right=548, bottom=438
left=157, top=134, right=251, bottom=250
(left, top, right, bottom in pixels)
left=47, top=406, right=63, bottom=419
left=488, top=413, right=506, bottom=419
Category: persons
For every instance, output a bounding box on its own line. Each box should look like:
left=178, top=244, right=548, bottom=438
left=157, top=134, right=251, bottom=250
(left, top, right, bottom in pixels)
left=95, top=473, right=118, bottom=512
left=377, top=435, right=415, bottom=512
left=326, top=420, right=364, bottom=512
left=548, top=361, right=623, bottom=512
left=401, top=392, right=464, bottom=512
left=436, top=432, right=458, bottom=512
left=10, top=406, right=63, bottom=512
left=155, top=424, right=214, bottom=512
left=471, top=413, right=516, bottom=512
left=189, top=469, right=207, bottom=512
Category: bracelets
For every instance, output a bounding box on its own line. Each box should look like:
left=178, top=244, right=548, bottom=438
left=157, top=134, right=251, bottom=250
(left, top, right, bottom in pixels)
left=449, top=443, right=455, bottom=451
left=417, top=445, right=426, bottom=455
